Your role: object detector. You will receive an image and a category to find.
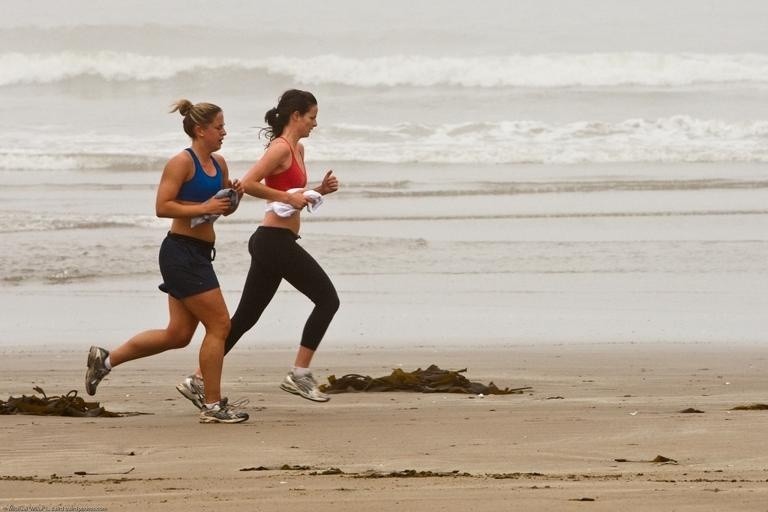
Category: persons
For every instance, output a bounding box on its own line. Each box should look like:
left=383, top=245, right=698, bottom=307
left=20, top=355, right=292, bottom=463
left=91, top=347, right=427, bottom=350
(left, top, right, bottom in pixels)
left=176, top=90, right=339, bottom=410
left=85, top=99, right=250, bottom=424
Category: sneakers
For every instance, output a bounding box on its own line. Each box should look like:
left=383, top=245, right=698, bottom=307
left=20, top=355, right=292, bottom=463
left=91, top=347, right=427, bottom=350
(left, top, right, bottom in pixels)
left=280, top=372, right=331, bottom=403
left=176, top=374, right=205, bottom=408
left=199, top=399, right=250, bottom=423
left=85, top=344, right=110, bottom=396
